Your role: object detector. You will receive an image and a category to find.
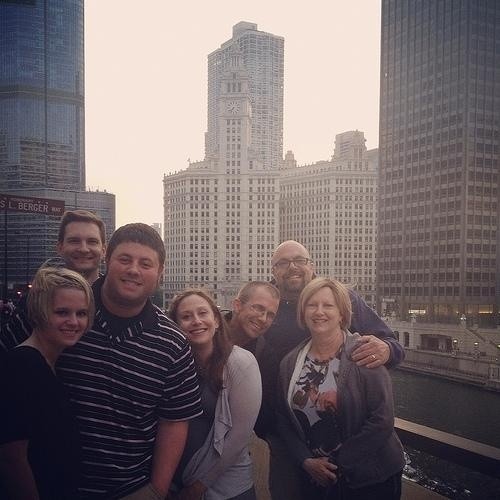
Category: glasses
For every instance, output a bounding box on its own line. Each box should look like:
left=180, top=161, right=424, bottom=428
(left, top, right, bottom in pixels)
left=273, top=257, right=313, bottom=269
left=241, top=299, right=275, bottom=322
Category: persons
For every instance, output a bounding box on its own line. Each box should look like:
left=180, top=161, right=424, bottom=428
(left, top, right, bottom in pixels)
left=266, top=240, right=404, bottom=499
left=54, top=222, right=203, bottom=499
left=0, top=211, right=105, bottom=350
left=221, top=281, right=282, bottom=499
left=0, top=267, right=93, bottom=499
left=278, top=277, right=405, bottom=499
left=168, top=290, right=262, bottom=499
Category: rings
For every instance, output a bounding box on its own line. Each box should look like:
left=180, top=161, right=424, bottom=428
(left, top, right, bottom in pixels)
left=372, top=355, right=377, bottom=361
